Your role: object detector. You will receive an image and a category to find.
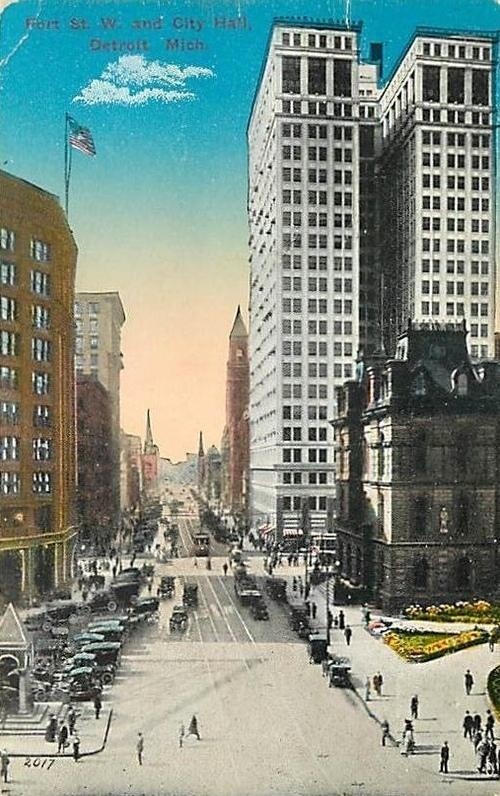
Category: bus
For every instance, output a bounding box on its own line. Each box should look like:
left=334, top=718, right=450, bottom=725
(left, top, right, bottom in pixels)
left=193, top=532, right=211, bottom=556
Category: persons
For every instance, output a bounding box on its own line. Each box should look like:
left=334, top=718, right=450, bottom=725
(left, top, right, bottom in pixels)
left=219, top=519, right=499, bottom=779
left=92, top=684, right=102, bottom=721
left=57, top=720, right=69, bottom=754
left=176, top=718, right=186, bottom=749
left=136, top=730, right=145, bottom=767
left=68, top=706, right=77, bottom=736
left=46, top=712, right=59, bottom=744
left=1, top=746, right=12, bottom=784
left=187, top=715, right=203, bottom=741
left=71, top=731, right=82, bottom=763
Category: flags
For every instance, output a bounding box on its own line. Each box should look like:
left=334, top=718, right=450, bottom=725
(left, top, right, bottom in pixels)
left=66, top=113, right=97, bottom=161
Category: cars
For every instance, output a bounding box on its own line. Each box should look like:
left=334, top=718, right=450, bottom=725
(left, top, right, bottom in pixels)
left=234, top=575, right=350, bottom=687
left=40, top=568, right=198, bottom=700
